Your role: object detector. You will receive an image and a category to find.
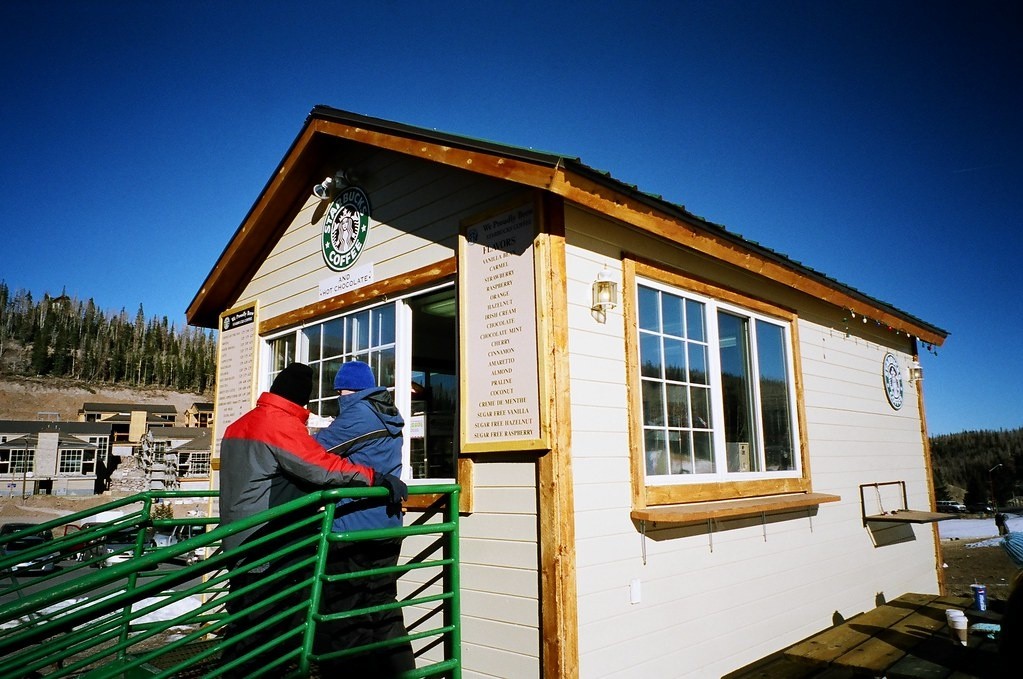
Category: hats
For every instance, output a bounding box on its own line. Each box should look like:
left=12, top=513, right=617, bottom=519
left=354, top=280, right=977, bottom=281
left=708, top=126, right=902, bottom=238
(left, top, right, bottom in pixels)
left=334, top=361, right=376, bottom=390
left=269, top=362, right=314, bottom=407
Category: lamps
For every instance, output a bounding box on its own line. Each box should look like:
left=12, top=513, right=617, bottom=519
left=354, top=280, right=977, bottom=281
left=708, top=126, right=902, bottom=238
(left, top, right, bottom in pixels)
left=909, top=356, right=924, bottom=381
left=591, top=264, right=618, bottom=317
left=314, top=172, right=341, bottom=199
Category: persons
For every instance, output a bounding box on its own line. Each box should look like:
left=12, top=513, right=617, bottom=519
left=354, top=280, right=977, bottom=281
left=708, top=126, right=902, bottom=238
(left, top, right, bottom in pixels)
left=219, top=360, right=417, bottom=679
left=995, top=511, right=1005, bottom=535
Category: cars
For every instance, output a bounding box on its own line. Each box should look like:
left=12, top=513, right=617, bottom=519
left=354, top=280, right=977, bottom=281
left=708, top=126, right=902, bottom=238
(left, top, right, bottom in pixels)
left=150, top=521, right=206, bottom=567
left=936, top=501, right=966, bottom=514
left=0, top=522, right=59, bottom=574
left=62, top=521, right=157, bottom=572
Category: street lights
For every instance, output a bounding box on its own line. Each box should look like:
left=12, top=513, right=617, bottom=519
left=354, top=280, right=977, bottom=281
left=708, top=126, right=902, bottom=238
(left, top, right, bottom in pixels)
left=989, top=463, right=1003, bottom=500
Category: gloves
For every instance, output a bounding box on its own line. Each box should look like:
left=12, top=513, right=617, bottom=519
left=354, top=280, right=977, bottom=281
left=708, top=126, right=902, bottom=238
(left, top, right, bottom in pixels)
left=374, top=472, right=408, bottom=505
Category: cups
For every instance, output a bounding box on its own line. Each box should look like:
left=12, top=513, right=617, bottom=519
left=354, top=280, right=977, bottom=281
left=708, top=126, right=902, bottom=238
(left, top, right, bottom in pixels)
left=970, top=584, right=987, bottom=613
left=946, top=609, right=964, bottom=626
left=949, top=614, right=968, bottom=646
